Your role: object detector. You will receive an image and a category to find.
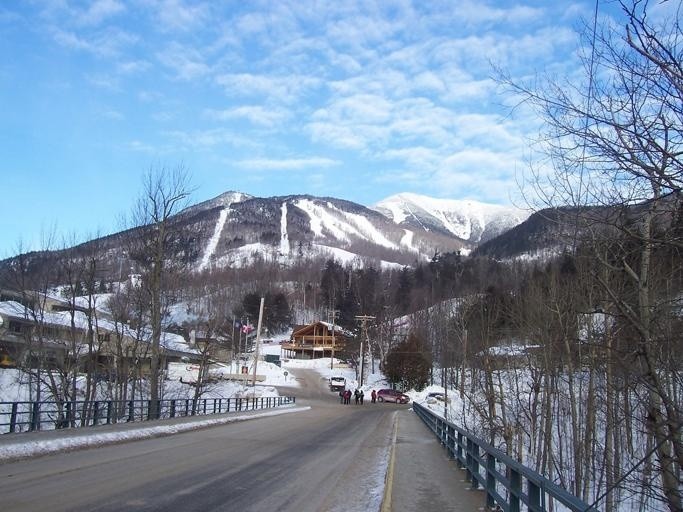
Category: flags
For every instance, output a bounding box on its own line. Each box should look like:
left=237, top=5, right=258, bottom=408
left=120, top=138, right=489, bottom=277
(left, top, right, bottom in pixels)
left=233, top=318, right=242, bottom=329
left=250, top=321, right=258, bottom=331
left=241, top=323, right=250, bottom=334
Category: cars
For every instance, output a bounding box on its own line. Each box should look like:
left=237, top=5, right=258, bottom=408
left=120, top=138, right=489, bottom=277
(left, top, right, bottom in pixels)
left=329, top=376, right=346, bottom=392
left=376, top=388, right=410, bottom=404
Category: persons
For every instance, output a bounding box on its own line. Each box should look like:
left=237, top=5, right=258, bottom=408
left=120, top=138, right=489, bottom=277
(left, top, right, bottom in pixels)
left=359, top=390, right=364, bottom=405
left=370, top=390, right=376, bottom=404
left=355, top=390, right=359, bottom=405
left=347, top=388, right=352, bottom=404
left=337, top=388, right=344, bottom=404
left=342, top=391, right=349, bottom=405
left=353, top=388, right=359, bottom=395
